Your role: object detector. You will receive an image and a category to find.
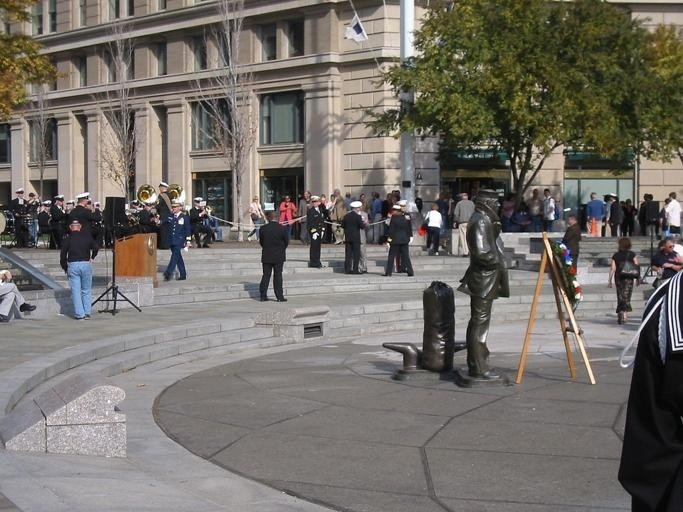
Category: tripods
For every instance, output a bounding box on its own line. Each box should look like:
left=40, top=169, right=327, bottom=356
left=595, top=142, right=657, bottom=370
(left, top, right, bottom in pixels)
left=91, top=229, right=145, bottom=315
left=640, top=225, right=664, bottom=279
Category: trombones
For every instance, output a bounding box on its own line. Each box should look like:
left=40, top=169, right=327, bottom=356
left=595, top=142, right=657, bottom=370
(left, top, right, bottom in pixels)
left=203, top=206, right=214, bottom=222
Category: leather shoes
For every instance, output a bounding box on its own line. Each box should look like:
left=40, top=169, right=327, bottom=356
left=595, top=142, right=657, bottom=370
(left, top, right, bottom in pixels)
left=164, top=270, right=170, bottom=280
left=0, top=314, right=8, bottom=322
left=176, top=277, right=187, bottom=280
left=20, top=303, right=36, bottom=312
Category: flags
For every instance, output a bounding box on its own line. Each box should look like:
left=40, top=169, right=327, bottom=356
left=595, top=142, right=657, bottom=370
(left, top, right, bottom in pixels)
left=343, top=14, right=369, bottom=43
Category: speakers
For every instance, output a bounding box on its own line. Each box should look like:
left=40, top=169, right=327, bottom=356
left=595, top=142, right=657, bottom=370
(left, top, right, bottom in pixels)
left=645, top=202, right=659, bottom=224
left=105, top=198, right=127, bottom=229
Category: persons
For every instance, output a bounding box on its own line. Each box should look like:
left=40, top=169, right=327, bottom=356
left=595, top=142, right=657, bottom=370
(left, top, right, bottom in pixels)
left=616, top=266, right=683, bottom=512
left=1, top=180, right=223, bottom=321
left=258, top=213, right=288, bottom=302
left=457, top=189, right=512, bottom=385
left=562, top=193, right=683, bottom=319
left=249, top=189, right=556, bottom=277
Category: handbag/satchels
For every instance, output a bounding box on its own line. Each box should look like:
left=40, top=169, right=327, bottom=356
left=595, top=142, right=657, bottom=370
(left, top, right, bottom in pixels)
left=419, top=224, right=427, bottom=235
left=616, top=260, right=639, bottom=278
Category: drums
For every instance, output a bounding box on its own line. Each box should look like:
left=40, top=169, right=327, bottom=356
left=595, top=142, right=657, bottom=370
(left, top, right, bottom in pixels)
left=0, top=209, right=15, bottom=236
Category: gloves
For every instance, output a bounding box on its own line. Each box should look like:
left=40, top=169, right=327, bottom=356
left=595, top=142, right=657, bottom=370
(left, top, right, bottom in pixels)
left=408, top=237, right=413, bottom=245
left=312, top=232, right=320, bottom=240
left=386, top=242, right=390, bottom=247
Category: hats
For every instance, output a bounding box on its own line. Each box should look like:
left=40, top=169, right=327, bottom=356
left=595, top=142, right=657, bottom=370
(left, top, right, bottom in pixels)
left=70, top=218, right=82, bottom=225
left=311, top=195, right=321, bottom=202
left=391, top=205, right=403, bottom=210
left=15, top=188, right=89, bottom=206
left=395, top=200, right=408, bottom=207
left=125, top=182, right=203, bottom=207
left=349, top=200, right=362, bottom=208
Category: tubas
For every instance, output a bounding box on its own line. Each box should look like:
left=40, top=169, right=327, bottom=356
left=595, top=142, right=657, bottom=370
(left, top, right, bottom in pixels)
left=136, top=185, right=157, bottom=207
left=166, top=185, right=185, bottom=205
